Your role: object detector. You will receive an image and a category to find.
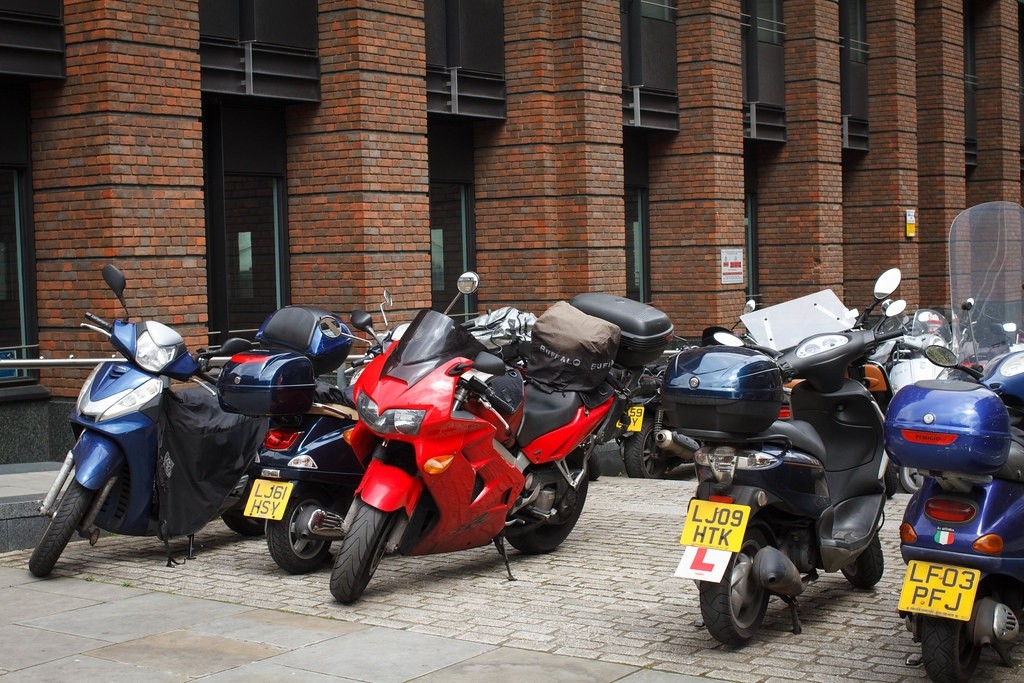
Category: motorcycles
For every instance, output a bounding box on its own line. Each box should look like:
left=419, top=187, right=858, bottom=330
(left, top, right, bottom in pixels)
left=29, top=257, right=353, bottom=579
left=660, top=299, right=908, bottom=646
left=215, top=271, right=539, bottom=574
left=613, top=299, right=756, bottom=479
left=329, top=271, right=674, bottom=605
left=667, top=267, right=1023, bottom=496
left=882, top=201, right=1022, bottom=683
